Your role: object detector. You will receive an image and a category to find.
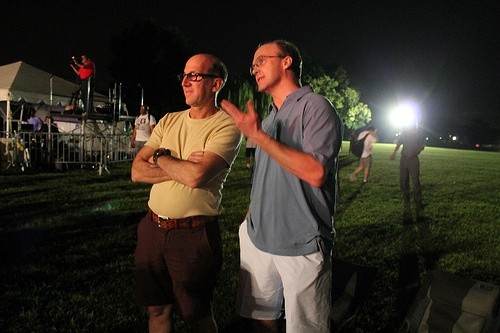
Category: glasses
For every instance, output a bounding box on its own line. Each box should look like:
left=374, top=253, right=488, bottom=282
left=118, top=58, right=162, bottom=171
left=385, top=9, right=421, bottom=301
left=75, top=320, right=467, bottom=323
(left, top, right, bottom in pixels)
left=177, top=72, right=220, bottom=82
left=250, top=54, right=285, bottom=75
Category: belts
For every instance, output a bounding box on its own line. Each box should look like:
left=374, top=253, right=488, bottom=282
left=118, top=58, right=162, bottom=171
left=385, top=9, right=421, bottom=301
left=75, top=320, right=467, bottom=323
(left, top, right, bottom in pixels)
left=149, top=208, right=217, bottom=230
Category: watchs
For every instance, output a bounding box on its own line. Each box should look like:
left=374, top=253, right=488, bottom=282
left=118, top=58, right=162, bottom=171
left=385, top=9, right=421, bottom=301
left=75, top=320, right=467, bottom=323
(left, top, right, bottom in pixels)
left=152, top=148, right=171, bottom=163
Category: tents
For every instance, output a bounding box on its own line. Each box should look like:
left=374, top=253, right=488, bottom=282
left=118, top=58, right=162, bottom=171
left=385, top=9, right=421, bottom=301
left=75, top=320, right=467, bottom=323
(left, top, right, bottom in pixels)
left=0, top=61, right=127, bottom=154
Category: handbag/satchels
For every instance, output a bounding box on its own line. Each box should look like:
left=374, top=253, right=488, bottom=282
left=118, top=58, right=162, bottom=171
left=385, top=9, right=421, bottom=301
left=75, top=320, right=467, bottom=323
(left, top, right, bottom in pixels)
left=349, top=139, right=365, bottom=158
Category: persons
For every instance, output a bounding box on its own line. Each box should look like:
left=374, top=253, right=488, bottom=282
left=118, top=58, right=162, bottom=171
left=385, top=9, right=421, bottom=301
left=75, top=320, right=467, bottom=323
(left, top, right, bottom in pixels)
left=391, top=127, right=426, bottom=221
left=348, top=125, right=377, bottom=183
left=130, top=53, right=244, bottom=333
left=132, top=104, right=156, bottom=153
left=70, top=55, right=95, bottom=114
left=220, top=41, right=344, bottom=333
left=41, top=116, right=59, bottom=161
